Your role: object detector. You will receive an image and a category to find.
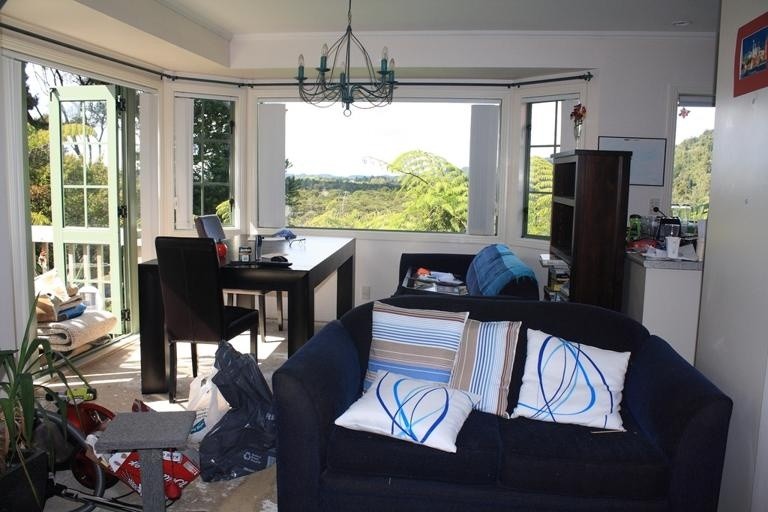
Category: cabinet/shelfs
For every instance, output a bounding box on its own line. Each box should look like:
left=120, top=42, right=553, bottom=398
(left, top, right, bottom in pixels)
left=547, top=149, right=633, bottom=313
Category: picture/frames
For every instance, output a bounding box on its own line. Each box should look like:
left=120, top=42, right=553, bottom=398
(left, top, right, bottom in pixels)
left=733, top=11, right=768, bottom=98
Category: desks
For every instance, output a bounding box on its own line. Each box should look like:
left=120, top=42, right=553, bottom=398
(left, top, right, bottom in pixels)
left=622, top=253, right=704, bottom=367
left=137, top=233, right=356, bottom=394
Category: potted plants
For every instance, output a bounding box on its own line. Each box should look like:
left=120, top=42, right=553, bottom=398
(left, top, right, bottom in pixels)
left=0, top=291, right=95, bottom=512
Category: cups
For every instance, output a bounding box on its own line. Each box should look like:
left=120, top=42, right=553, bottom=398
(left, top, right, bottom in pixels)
left=641, top=216, right=657, bottom=238
left=665, top=237, right=681, bottom=258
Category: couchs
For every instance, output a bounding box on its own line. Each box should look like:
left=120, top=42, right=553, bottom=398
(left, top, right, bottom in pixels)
left=390, top=242, right=539, bottom=297
left=272, top=297, right=734, bottom=512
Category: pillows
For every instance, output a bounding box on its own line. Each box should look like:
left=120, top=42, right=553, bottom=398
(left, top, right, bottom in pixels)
left=334, top=369, right=480, bottom=454
left=450, top=319, right=522, bottom=420
left=362, top=301, right=470, bottom=396
left=511, top=328, right=631, bottom=433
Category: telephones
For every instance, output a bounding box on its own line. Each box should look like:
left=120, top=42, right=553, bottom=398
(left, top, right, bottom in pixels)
left=255, top=235, right=265, bottom=265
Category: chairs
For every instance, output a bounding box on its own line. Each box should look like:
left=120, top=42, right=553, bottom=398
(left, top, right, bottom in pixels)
left=194, top=214, right=283, bottom=344
left=155, top=236, right=260, bottom=403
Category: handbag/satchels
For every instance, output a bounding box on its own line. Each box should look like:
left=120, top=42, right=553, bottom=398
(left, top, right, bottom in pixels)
left=186, top=339, right=276, bottom=481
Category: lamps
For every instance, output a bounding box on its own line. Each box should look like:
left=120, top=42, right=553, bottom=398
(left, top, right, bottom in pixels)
left=295, top=0, right=398, bottom=117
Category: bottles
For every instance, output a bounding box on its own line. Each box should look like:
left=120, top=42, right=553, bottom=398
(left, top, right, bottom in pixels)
left=255, top=234, right=264, bottom=260
left=686, top=208, right=698, bottom=238
left=628, top=214, right=641, bottom=241
left=239, top=247, right=251, bottom=263
left=670, top=203, right=680, bottom=218
left=679, top=204, right=691, bottom=231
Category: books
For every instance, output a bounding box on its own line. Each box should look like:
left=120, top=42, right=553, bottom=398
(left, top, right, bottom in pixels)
left=539, top=253, right=570, bottom=303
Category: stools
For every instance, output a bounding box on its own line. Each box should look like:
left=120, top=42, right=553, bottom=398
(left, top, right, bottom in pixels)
left=94, top=412, right=197, bottom=511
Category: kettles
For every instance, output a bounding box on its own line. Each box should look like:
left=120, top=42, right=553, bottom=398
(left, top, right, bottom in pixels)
left=657, top=216, right=681, bottom=245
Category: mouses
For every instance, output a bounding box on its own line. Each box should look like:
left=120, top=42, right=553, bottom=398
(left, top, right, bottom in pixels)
left=271, top=256, right=287, bottom=262
